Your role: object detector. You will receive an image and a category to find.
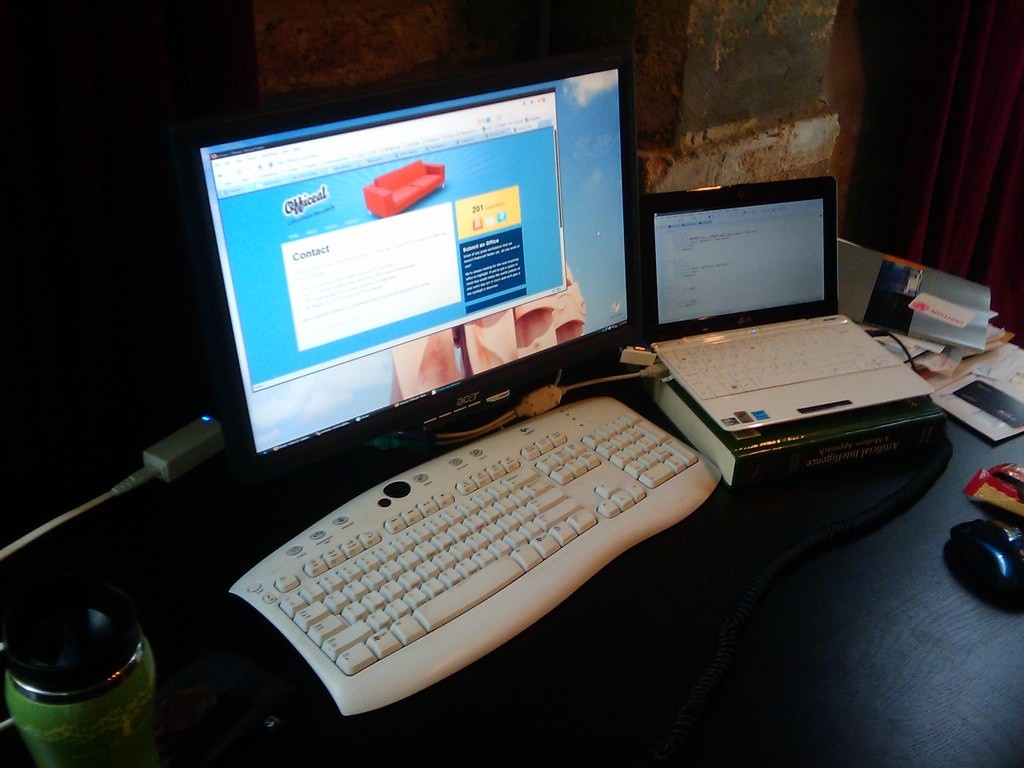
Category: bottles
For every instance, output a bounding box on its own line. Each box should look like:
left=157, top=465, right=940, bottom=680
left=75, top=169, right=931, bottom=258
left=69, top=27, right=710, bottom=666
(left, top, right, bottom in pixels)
left=0, top=586, right=161, bottom=768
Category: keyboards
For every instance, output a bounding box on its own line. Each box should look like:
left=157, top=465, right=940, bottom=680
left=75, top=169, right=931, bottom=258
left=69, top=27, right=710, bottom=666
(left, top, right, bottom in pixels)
left=224, top=395, right=727, bottom=719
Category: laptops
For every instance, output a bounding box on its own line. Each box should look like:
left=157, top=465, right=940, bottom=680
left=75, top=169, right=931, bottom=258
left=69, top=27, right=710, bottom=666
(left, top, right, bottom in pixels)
left=650, top=175, right=935, bottom=434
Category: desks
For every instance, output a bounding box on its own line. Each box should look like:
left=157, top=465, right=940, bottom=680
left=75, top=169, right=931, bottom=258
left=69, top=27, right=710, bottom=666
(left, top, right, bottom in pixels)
left=0, top=235, right=1024, bottom=768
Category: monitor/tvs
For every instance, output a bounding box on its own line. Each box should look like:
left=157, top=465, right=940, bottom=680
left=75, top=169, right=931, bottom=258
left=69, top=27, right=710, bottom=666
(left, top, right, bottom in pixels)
left=174, top=41, right=649, bottom=503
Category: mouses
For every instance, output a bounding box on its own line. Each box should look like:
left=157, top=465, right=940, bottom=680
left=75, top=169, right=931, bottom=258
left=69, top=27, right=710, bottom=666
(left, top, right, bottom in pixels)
left=945, top=519, right=1024, bottom=613
